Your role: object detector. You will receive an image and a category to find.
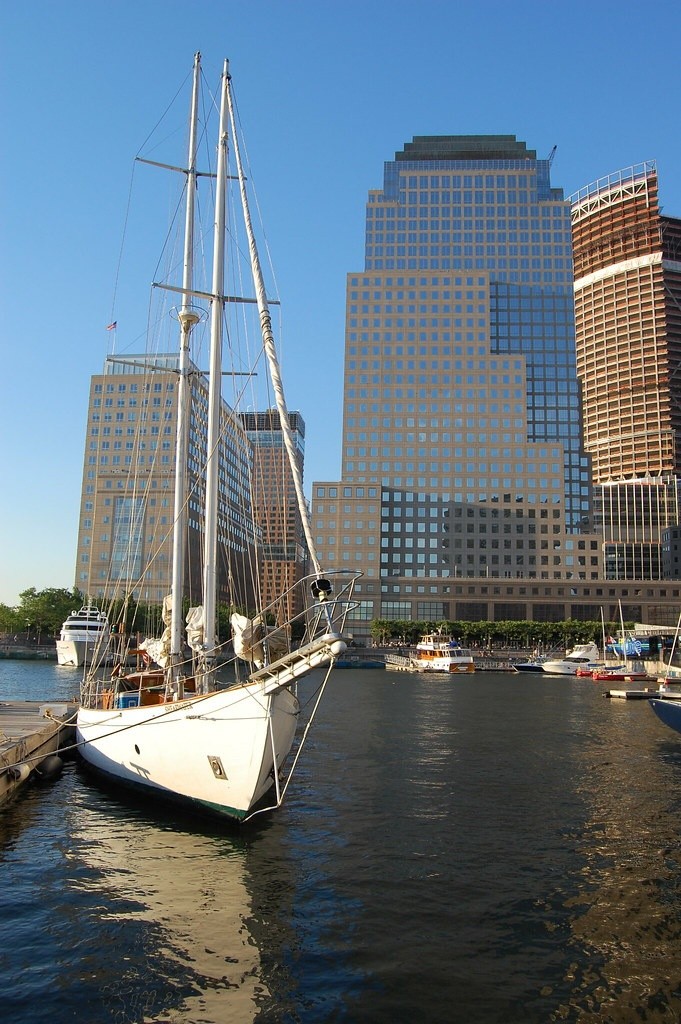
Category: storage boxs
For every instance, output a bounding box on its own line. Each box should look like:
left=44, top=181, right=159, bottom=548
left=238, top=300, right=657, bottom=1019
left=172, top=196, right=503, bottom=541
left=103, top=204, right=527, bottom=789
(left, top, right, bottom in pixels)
left=114, top=692, right=142, bottom=709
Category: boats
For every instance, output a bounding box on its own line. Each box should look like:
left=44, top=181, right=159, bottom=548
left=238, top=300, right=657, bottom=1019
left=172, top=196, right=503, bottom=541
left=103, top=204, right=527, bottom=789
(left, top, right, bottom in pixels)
left=383, top=623, right=476, bottom=675
left=55, top=591, right=115, bottom=667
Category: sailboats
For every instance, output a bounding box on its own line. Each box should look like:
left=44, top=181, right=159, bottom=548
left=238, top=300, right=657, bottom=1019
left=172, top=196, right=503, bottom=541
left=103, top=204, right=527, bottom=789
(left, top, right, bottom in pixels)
left=69, top=47, right=367, bottom=827
left=511, top=598, right=681, bottom=734
